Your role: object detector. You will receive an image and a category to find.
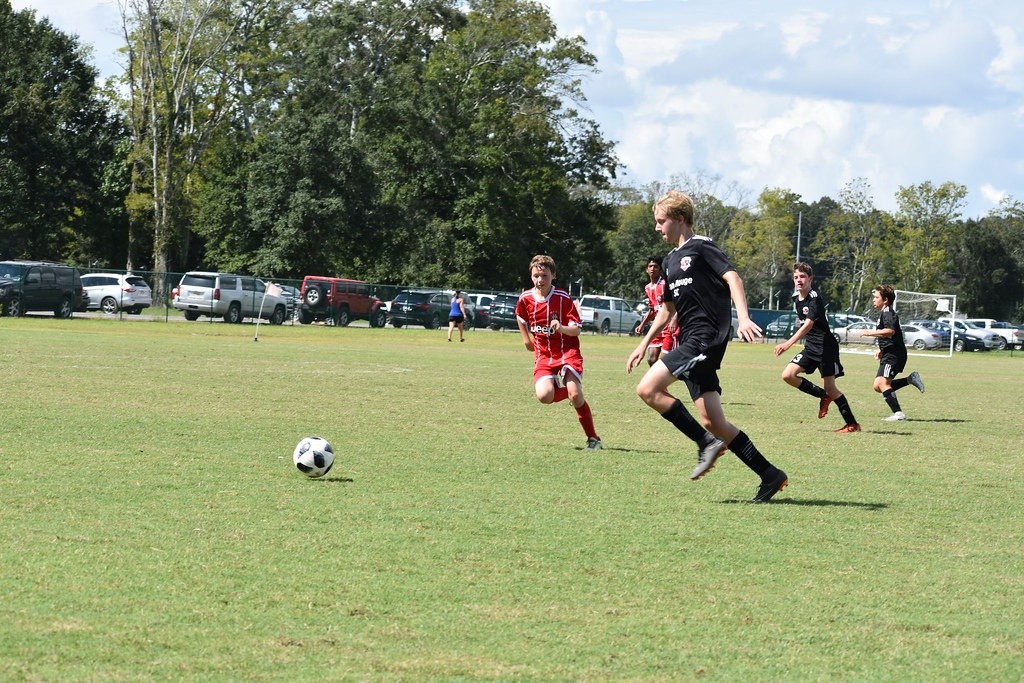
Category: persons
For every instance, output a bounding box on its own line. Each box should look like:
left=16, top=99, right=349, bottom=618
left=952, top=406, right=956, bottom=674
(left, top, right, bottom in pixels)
left=447, top=291, right=466, bottom=342
left=774, top=263, right=861, bottom=433
left=860, top=285, right=924, bottom=422
left=634, top=257, right=680, bottom=368
left=626, top=191, right=789, bottom=504
left=516, top=255, right=603, bottom=451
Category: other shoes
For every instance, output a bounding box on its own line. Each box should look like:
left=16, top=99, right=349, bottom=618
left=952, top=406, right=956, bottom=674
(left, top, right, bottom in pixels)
left=586, top=437, right=603, bottom=451
left=460, top=339, right=464, bottom=342
left=448, top=339, right=451, bottom=342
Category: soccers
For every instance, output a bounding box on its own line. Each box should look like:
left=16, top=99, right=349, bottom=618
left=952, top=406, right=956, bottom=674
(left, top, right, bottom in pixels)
left=292, top=435, right=334, bottom=478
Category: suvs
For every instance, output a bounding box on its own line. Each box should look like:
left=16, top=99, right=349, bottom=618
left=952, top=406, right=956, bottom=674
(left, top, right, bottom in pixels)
left=0, top=259, right=84, bottom=319
left=80, top=272, right=152, bottom=315
left=298, top=275, right=386, bottom=327
left=171, top=270, right=288, bottom=325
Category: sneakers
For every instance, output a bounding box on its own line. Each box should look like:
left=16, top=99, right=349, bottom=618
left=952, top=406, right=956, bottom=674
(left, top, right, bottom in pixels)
left=835, top=424, right=861, bottom=434
left=690, top=437, right=727, bottom=480
left=910, top=371, right=925, bottom=393
left=818, top=395, right=832, bottom=418
left=752, top=469, right=788, bottom=504
left=881, top=412, right=906, bottom=421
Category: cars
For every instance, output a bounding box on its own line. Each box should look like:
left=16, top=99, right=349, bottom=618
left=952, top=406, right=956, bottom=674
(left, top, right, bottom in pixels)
left=389, top=287, right=1023, bottom=351
